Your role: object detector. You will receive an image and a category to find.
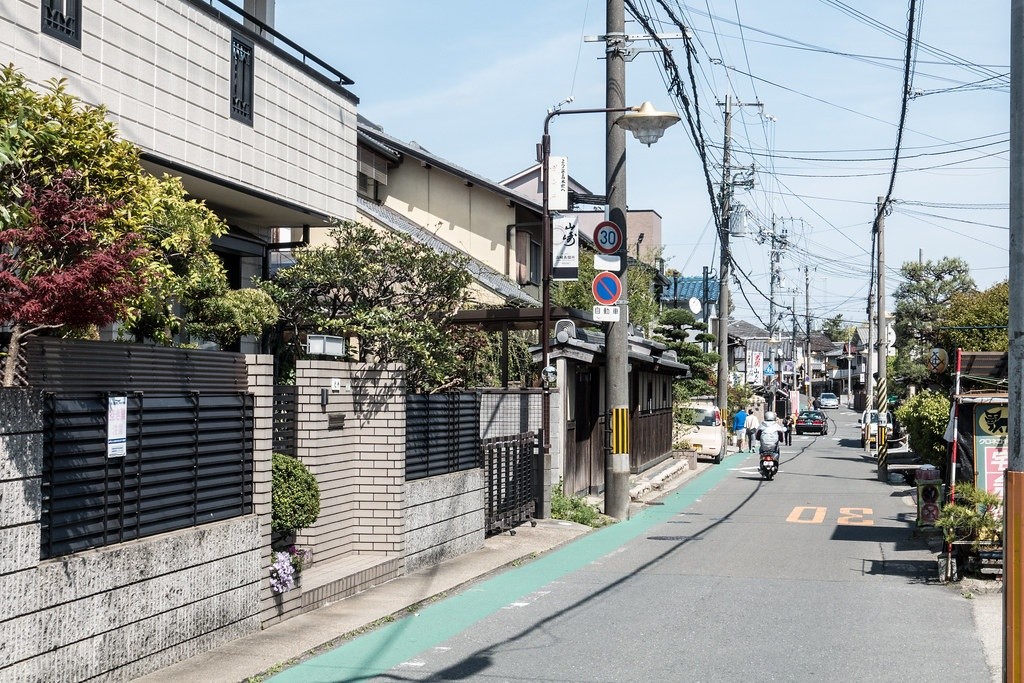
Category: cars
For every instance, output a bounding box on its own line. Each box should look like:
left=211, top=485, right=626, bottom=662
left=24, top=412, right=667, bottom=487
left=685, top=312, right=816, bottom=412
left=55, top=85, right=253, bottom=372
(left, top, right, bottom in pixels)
left=795, top=410, right=828, bottom=436
left=818, top=393, right=840, bottom=409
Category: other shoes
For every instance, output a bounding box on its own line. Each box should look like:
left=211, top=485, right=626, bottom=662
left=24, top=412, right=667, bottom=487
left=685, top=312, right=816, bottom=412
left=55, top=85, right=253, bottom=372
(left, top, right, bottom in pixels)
left=752, top=447, right=756, bottom=453
left=789, top=444, right=792, bottom=446
left=749, top=450, right=751, bottom=453
left=739, top=448, right=744, bottom=453
left=786, top=443, right=787, bottom=445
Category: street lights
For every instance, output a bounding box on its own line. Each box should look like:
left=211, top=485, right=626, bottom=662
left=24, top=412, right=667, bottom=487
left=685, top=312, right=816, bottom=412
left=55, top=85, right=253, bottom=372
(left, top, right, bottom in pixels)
left=535, top=101, right=682, bottom=454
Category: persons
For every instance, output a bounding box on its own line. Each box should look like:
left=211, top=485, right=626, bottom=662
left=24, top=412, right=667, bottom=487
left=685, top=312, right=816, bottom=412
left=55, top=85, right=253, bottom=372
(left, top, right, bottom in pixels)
left=732, top=406, right=794, bottom=457
left=812, top=396, right=822, bottom=410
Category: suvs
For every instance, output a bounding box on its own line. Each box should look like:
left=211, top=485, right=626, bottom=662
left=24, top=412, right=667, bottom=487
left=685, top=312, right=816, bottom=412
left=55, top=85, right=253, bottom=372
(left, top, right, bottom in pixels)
left=672, top=403, right=727, bottom=464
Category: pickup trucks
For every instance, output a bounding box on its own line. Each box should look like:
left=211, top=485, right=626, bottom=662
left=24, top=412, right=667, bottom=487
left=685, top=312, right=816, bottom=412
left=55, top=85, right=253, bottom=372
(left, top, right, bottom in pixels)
left=858, top=409, right=896, bottom=449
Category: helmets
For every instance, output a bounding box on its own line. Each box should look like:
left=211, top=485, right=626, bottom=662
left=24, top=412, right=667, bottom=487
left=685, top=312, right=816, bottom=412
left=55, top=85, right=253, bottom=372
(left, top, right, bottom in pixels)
left=765, top=411, right=776, bottom=420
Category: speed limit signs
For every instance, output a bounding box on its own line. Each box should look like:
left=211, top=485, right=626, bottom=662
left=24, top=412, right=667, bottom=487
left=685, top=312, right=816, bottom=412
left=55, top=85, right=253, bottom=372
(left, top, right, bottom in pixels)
left=593, top=221, right=623, bottom=255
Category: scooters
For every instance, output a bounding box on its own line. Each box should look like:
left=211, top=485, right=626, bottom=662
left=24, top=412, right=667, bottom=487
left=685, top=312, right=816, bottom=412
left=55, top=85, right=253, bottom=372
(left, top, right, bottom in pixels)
left=754, top=425, right=787, bottom=481
